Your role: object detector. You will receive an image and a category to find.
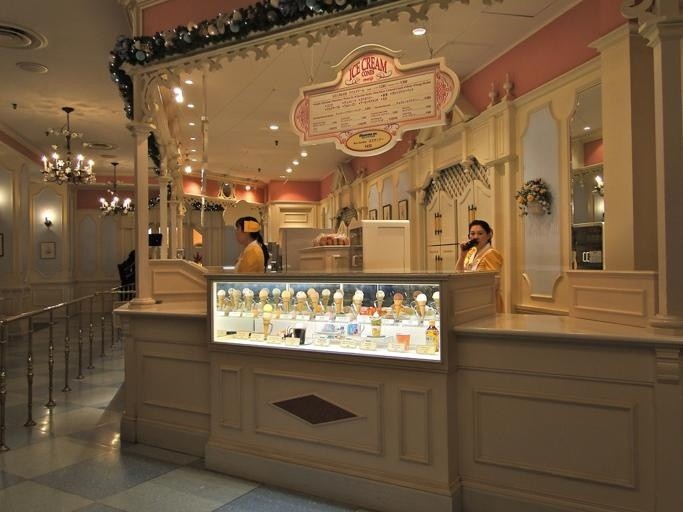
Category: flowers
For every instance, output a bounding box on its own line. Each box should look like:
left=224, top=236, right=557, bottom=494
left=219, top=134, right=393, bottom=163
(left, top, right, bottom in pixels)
left=513, top=177, right=551, bottom=218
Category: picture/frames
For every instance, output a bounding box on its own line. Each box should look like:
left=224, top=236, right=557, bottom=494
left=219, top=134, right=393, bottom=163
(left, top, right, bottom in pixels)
left=40, top=242, right=57, bottom=259
left=397, top=200, right=408, bottom=220
left=369, top=209, right=377, bottom=220
left=0, top=233, right=4, bottom=257
left=382, top=204, right=392, bottom=220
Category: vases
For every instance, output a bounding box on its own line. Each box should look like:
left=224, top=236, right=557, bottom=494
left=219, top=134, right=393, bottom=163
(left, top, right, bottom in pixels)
left=525, top=200, right=545, bottom=216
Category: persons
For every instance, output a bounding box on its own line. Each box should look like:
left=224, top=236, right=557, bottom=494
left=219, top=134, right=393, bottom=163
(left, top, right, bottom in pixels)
left=234, top=216, right=270, bottom=273
left=455, top=219, right=505, bottom=312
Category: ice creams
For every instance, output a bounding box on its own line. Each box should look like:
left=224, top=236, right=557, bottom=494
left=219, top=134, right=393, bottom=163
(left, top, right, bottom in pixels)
left=216, top=288, right=441, bottom=342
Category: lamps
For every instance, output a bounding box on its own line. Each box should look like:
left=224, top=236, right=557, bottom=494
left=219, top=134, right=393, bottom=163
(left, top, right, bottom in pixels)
left=41, top=107, right=135, bottom=217
left=45, top=217, right=51, bottom=226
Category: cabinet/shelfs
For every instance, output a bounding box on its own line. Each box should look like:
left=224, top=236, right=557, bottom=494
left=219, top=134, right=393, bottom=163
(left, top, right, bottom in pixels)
left=201, top=268, right=497, bottom=372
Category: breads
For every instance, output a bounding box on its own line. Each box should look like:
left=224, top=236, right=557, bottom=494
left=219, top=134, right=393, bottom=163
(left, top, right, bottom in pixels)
left=311, top=233, right=350, bottom=246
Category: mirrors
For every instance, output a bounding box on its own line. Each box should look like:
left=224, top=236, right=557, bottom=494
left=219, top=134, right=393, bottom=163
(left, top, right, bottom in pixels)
left=564, top=78, right=606, bottom=270
